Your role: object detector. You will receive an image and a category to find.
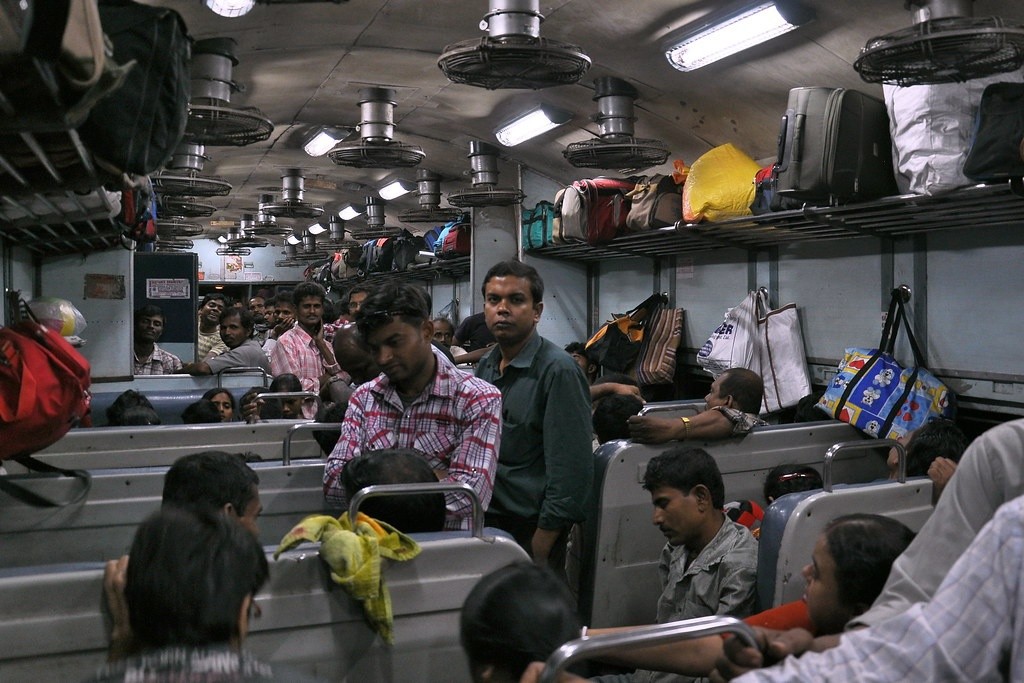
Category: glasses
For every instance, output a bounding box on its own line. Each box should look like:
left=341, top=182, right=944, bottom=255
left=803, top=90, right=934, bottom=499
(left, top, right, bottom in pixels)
left=356, top=310, right=410, bottom=334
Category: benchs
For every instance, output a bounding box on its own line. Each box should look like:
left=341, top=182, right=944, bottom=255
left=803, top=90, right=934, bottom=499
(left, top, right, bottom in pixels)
left=0, top=366, right=946, bottom=683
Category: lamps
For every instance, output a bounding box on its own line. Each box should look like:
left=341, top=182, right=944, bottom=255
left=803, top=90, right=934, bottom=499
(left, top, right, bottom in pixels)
left=205, top=0, right=255, bottom=18
left=660, top=0, right=818, bottom=72
left=286, top=232, right=303, bottom=246
left=494, top=103, right=577, bottom=147
left=338, top=203, right=367, bottom=221
left=304, top=125, right=350, bottom=158
left=378, top=178, right=417, bottom=200
left=308, top=223, right=330, bottom=235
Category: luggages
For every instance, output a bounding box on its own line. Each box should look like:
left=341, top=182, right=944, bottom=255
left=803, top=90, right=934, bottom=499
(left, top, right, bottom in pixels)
left=778, top=87, right=890, bottom=206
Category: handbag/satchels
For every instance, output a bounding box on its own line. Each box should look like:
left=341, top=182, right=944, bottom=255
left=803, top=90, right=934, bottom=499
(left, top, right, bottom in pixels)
left=0, top=291, right=91, bottom=462
left=520, top=145, right=780, bottom=249
left=963, top=81, right=1024, bottom=185
left=584, top=292, right=686, bottom=385
left=696, top=290, right=812, bottom=415
left=302, top=224, right=470, bottom=283
left=0, top=0, right=189, bottom=238
left=812, top=287, right=951, bottom=440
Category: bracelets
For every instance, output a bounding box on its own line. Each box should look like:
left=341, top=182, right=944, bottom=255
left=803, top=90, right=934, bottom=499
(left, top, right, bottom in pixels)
left=581, top=626, right=591, bottom=638
left=681, top=416, right=691, bottom=442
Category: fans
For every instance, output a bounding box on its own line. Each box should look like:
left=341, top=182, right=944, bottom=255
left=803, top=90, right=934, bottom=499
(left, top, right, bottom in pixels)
left=145, top=125, right=461, bottom=268
left=563, top=75, right=674, bottom=172
left=439, top=0, right=589, bottom=91
left=444, top=143, right=527, bottom=205
left=854, top=0, right=1024, bottom=86
left=325, top=89, right=426, bottom=168
left=178, top=37, right=273, bottom=148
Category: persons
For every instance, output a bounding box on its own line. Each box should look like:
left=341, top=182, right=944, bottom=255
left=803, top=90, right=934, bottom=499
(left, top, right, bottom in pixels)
left=96, top=258, right=1024, bottom=683
left=474, top=264, right=592, bottom=573
